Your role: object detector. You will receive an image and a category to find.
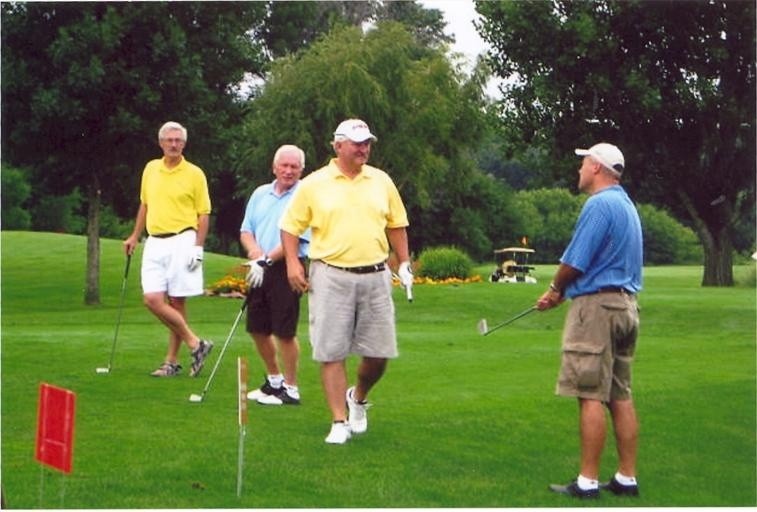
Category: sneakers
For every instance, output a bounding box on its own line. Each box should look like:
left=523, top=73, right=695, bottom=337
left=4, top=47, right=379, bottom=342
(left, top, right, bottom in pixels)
left=549, top=479, right=600, bottom=500
left=246, top=375, right=284, bottom=401
left=324, top=420, right=350, bottom=445
left=345, top=385, right=369, bottom=435
left=257, top=384, right=300, bottom=404
left=597, top=477, right=640, bottom=499
left=189, top=339, right=214, bottom=377
left=149, top=362, right=184, bottom=378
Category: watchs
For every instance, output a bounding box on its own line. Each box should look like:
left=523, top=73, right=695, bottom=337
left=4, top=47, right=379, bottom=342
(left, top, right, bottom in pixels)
left=263, top=255, right=274, bottom=267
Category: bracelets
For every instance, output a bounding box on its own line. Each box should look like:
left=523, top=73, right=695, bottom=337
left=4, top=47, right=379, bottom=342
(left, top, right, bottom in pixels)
left=549, top=282, right=559, bottom=294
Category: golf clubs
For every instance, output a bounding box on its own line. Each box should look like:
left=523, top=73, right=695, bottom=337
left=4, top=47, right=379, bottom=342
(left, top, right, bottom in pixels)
left=477, top=306, right=539, bottom=335
left=188, top=287, right=246, bottom=402
left=95, top=255, right=132, bottom=373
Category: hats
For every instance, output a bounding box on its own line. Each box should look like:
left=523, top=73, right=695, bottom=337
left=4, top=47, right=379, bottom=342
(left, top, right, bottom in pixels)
left=574, top=142, right=624, bottom=179
left=334, top=117, right=378, bottom=145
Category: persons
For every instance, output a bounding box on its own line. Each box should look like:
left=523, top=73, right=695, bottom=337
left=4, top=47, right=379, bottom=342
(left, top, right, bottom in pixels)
left=278, top=118, right=415, bottom=444
left=532, top=141, right=644, bottom=499
left=238, top=143, right=313, bottom=406
left=122, top=121, right=216, bottom=379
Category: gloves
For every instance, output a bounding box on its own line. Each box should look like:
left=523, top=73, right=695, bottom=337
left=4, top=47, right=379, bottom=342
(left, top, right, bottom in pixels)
left=242, top=255, right=272, bottom=288
left=399, top=262, right=413, bottom=287
left=187, top=246, right=204, bottom=273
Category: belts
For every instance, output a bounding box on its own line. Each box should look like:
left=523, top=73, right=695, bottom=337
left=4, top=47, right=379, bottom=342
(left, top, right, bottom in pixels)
left=148, top=226, right=194, bottom=240
left=570, top=285, right=634, bottom=299
left=316, top=257, right=389, bottom=275
